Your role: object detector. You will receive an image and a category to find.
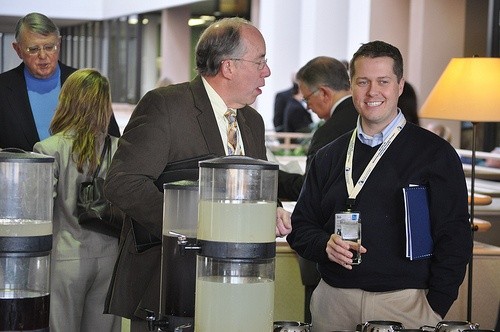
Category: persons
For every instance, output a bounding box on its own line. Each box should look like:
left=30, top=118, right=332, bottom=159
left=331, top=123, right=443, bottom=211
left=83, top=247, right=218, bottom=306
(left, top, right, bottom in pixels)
left=102, top=18, right=292, bottom=332
left=33, top=69, right=122, bottom=332
left=285, top=41, right=474, bottom=332
left=296, top=56, right=359, bottom=323
left=0, top=13, right=121, bottom=152
left=397, top=82, right=419, bottom=125
left=274, top=74, right=314, bottom=144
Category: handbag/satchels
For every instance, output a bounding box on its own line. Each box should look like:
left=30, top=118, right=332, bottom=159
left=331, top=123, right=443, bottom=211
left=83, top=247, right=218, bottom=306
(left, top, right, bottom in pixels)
left=77, top=176, right=124, bottom=238
left=132, top=154, right=221, bottom=255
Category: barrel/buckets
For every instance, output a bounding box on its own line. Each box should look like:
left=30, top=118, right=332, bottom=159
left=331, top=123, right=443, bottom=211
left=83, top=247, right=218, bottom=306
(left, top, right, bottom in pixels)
left=144, top=154, right=279, bottom=332
left=0, top=146, right=57, bottom=331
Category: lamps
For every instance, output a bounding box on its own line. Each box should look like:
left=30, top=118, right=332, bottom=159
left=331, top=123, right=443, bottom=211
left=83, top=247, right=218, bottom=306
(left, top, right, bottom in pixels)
left=416, top=58, right=500, bottom=323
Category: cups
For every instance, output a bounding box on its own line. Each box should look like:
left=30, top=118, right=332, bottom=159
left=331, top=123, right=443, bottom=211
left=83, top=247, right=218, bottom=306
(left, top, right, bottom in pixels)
left=340, top=218, right=362, bottom=265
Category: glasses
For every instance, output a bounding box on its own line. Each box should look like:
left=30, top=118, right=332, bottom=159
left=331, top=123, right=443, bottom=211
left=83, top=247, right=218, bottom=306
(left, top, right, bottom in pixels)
left=302, top=88, right=321, bottom=103
left=220, top=58, right=268, bottom=71
left=17, top=37, right=59, bottom=55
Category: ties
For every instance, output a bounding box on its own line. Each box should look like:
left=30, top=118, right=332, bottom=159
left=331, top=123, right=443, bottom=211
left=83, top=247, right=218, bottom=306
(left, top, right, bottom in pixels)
left=224, top=108, right=243, bottom=156
